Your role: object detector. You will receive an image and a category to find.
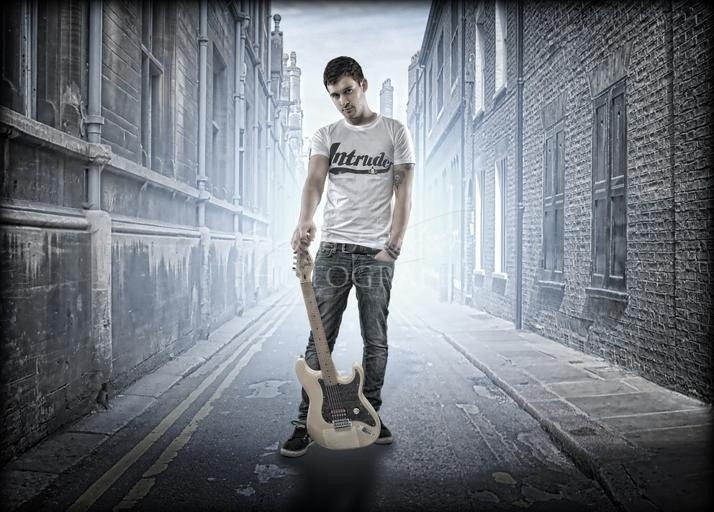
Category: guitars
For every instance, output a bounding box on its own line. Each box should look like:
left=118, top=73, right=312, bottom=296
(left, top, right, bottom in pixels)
left=292, top=233, right=381, bottom=450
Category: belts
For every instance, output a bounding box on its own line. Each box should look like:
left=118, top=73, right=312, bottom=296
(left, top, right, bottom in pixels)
left=319, top=241, right=383, bottom=254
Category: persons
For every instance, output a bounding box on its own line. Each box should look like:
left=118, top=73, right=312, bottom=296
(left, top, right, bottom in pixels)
left=276, top=55, right=413, bottom=456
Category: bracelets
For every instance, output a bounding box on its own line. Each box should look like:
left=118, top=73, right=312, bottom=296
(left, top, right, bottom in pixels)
left=384, top=241, right=401, bottom=258
left=382, top=246, right=398, bottom=261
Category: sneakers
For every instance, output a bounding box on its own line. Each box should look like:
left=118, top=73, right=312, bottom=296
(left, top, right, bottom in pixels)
left=280, top=418, right=317, bottom=458
left=374, top=416, right=393, bottom=446
